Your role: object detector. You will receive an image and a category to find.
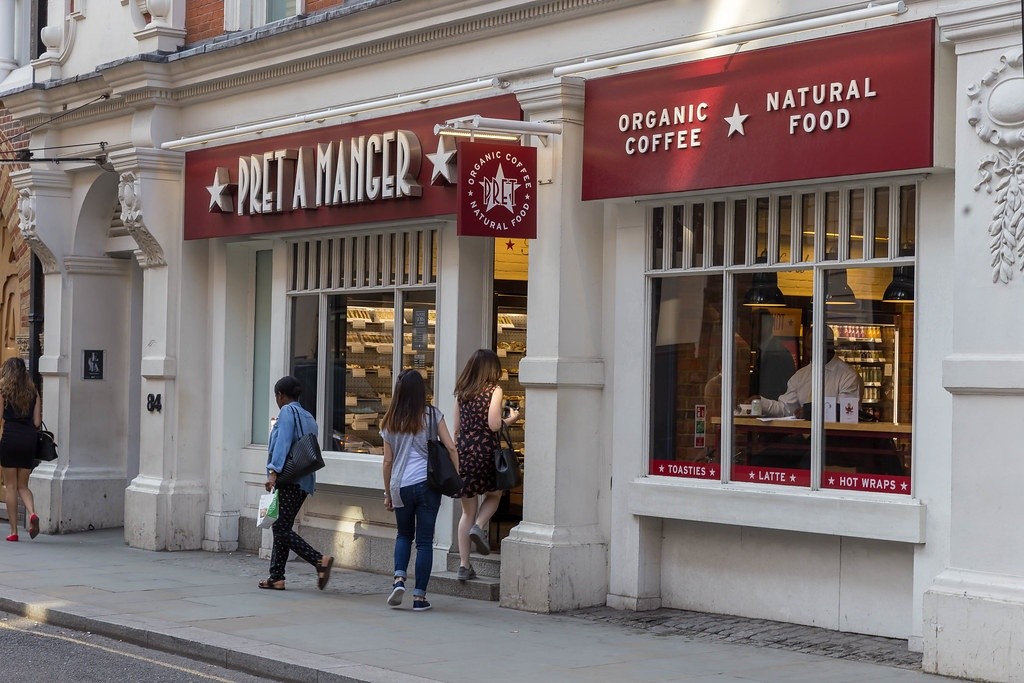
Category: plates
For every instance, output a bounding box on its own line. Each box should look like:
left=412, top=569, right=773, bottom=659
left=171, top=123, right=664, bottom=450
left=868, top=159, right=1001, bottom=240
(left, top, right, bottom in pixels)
left=735, top=414, right=767, bottom=417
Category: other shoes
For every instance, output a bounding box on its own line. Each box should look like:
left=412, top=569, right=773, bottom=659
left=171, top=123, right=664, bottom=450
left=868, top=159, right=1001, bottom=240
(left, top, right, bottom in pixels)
left=29, top=514, right=40, bottom=539
left=7, top=534, right=18, bottom=541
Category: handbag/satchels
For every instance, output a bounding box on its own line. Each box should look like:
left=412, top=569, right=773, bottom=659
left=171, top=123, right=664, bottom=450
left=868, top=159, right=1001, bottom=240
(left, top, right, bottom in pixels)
left=35, top=419, right=58, bottom=461
left=427, top=405, right=463, bottom=497
left=256, top=486, right=279, bottom=527
left=488, top=422, right=522, bottom=489
left=280, top=405, right=326, bottom=481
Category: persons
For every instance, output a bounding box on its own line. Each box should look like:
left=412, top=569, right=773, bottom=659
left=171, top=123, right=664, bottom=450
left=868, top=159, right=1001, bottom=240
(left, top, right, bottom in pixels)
left=749, top=308, right=796, bottom=401
left=0, top=358, right=40, bottom=541
left=258, top=376, right=334, bottom=591
left=379, top=369, right=459, bottom=611
left=745, top=325, right=864, bottom=462
left=705, top=357, right=721, bottom=394
left=453, top=349, right=520, bottom=580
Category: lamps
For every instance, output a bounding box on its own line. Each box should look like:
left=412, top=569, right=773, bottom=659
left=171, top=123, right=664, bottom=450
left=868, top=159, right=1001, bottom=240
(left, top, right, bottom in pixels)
left=162, top=79, right=501, bottom=150
left=810, top=198, right=857, bottom=306
left=743, top=207, right=788, bottom=306
left=552, top=0, right=906, bottom=76
left=433, top=116, right=562, bottom=147
left=882, top=189, right=914, bottom=303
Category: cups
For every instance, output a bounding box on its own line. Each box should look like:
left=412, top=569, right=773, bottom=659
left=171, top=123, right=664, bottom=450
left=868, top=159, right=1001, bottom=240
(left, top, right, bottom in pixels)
left=739, top=404, right=752, bottom=414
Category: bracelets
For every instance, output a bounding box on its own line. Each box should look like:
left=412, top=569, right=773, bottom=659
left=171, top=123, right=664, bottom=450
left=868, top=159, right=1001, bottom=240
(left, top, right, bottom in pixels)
left=269, top=470, right=274, bottom=473
left=384, top=492, right=390, bottom=497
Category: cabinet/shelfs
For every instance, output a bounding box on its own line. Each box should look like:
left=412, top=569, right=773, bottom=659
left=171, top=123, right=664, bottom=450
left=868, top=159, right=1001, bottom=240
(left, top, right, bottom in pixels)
left=346, top=299, right=527, bottom=508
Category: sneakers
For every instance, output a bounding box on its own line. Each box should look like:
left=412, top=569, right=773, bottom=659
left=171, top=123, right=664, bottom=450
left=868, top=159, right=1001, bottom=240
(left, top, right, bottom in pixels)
left=413, top=600, right=432, bottom=611
left=458, top=565, right=475, bottom=580
left=387, top=582, right=405, bottom=606
left=469, top=524, right=490, bottom=555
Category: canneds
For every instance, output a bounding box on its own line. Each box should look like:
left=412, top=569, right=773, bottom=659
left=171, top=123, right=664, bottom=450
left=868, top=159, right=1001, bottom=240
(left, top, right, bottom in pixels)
left=863, top=387, right=881, bottom=400
left=751, top=399, right=762, bottom=415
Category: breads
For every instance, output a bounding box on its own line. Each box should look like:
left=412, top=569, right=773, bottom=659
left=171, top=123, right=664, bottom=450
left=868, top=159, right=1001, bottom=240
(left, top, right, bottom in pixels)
left=497, top=341, right=525, bottom=399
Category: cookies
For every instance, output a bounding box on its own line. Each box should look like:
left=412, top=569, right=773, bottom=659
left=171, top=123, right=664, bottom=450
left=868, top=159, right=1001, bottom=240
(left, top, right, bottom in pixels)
left=499, top=315, right=513, bottom=328
left=344, top=310, right=435, bottom=371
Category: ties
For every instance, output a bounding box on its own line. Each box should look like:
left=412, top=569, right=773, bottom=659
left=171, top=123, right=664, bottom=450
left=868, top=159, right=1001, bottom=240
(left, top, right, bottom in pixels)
left=756, top=348, right=762, bottom=369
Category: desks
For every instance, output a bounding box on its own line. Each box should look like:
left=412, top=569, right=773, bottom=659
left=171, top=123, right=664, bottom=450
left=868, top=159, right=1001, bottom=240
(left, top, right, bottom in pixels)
left=710, top=416, right=912, bottom=472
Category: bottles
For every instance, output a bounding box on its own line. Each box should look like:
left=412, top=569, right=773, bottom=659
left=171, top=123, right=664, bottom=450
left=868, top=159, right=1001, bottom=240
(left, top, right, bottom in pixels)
left=823, top=323, right=884, bottom=400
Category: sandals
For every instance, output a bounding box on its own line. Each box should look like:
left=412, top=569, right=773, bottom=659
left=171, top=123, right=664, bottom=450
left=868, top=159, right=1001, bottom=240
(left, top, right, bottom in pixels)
left=317, top=556, right=334, bottom=590
left=258, top=576, right=285, bottom=591
